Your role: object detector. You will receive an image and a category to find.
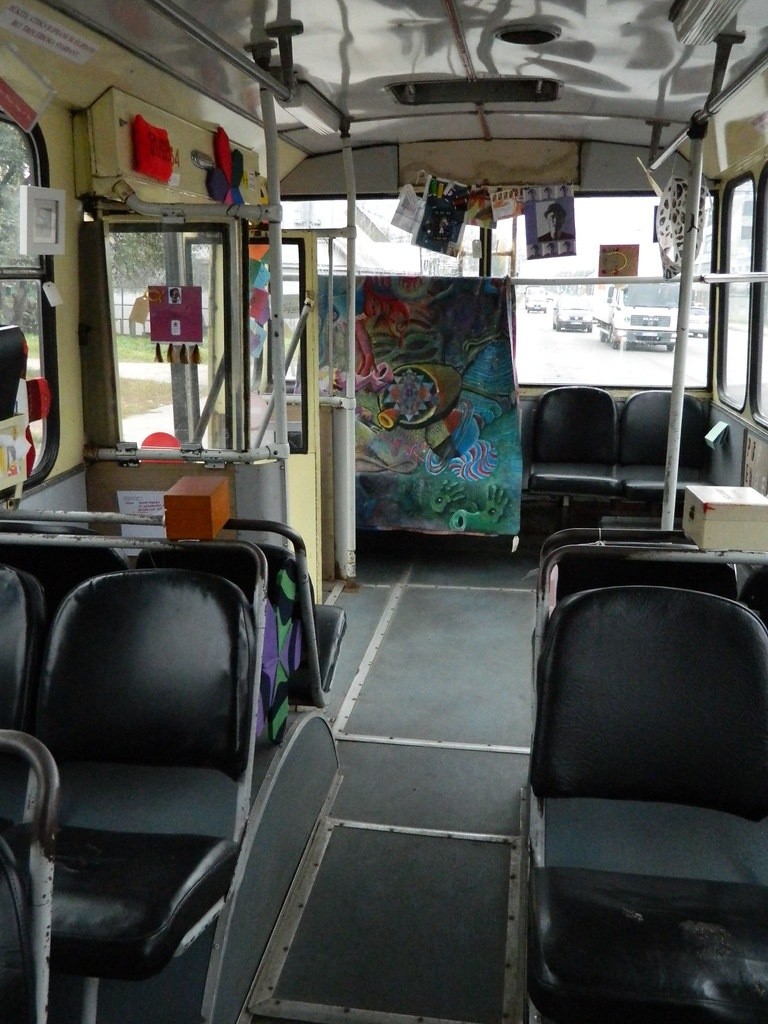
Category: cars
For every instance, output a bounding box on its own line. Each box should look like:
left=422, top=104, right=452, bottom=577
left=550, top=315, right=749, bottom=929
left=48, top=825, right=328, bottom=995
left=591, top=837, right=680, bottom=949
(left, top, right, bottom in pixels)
left=683, top=306, right=709, bottom=338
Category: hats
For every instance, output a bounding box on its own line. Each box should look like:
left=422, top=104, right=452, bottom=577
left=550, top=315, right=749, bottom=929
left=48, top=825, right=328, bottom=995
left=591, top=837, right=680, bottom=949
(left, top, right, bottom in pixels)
left=544, top=202, right=566, bottom=218
left=547, top=243, right=555, bottom=248
left=563, top=241, right=571, bottom=246
left=531, top=245, right=539, bottom=249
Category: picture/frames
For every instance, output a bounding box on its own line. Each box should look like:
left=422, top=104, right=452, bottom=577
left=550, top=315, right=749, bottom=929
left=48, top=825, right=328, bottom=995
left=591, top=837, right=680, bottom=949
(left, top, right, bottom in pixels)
left=19, top=180, right=68, bottom=263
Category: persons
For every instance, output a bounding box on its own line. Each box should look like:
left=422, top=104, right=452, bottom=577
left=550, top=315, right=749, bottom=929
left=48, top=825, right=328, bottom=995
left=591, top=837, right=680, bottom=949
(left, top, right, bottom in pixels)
left=527, top=245, right=543, bottom=259
left=560, top=241, right=576, bottom=256
left=538, top=203, right=575, bottom=242
left=556, top=185, right=574, bottom=200
left=543, top=243, right=559, bottom=257
left=540, top=187, right=556, bottom=203
left=526, top=189, right=539, bottom=203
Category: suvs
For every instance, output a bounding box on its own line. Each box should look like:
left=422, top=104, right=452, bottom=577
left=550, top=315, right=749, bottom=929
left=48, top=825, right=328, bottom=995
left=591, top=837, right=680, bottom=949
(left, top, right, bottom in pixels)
left=552, top=295, right=593, bottom=333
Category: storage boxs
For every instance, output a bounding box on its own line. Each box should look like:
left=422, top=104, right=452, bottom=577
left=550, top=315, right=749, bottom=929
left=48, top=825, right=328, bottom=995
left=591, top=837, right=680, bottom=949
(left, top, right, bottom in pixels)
left=681, top=484, right=768, bottom=557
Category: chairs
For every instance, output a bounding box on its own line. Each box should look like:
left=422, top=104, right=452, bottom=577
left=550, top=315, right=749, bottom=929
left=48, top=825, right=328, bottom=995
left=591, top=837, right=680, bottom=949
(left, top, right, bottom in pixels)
left=613, top=392, right=719, bottom=517
left=517, top=386, right=616, bottom=514
left=3, top=512, right=767, bottom=1024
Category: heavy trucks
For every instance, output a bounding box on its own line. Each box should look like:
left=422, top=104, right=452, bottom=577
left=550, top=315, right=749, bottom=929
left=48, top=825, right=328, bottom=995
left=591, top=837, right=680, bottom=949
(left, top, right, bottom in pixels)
left=595, top=282, right=677, bottom=353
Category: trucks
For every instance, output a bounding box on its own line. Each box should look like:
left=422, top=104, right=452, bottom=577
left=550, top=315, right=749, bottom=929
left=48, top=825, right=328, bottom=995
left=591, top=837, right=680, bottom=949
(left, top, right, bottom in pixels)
left=524, top=285, right=547, bottom=313
